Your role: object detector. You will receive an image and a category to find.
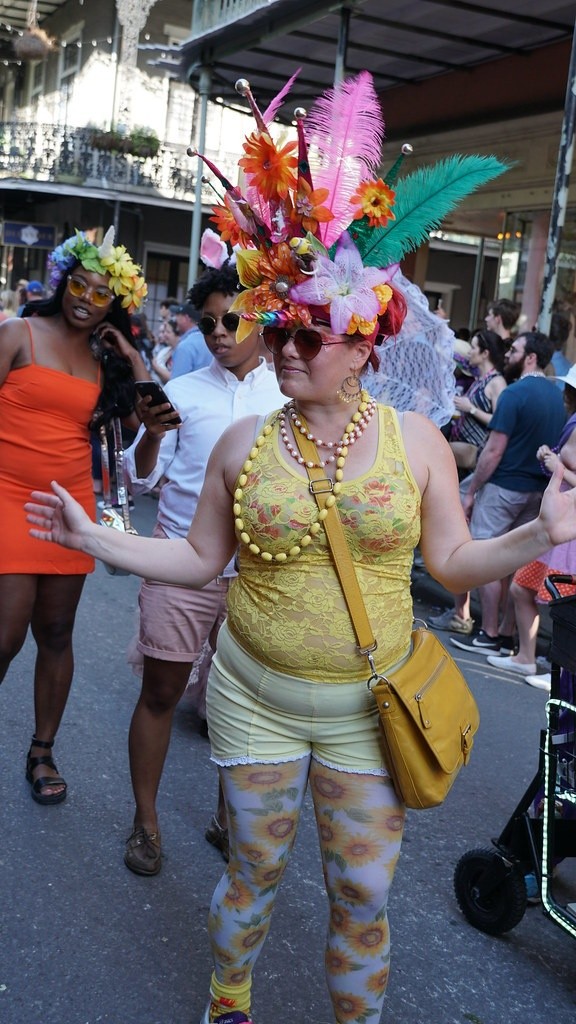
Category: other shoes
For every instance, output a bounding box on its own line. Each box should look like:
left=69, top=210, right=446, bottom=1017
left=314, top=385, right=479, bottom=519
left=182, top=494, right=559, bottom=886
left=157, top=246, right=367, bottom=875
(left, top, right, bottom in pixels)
left=201, top=999, right=253, bottom=1024
left=525, top=874, right=542, bottom=902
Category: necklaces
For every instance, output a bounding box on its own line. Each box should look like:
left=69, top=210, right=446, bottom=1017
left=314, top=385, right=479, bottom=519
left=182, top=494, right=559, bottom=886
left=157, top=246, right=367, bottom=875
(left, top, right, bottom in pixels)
left=234, top=389, right=375, bottom=564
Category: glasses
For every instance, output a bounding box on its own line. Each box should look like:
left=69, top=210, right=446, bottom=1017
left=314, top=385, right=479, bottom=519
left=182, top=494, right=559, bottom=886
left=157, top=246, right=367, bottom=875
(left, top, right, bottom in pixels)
left=195, top=311, right=240, bottom=335
left=66, top=272, right=116, bottom=307
left=508, top=346, right=526, bottom=359
left=258, top=323, right=361, bottom=361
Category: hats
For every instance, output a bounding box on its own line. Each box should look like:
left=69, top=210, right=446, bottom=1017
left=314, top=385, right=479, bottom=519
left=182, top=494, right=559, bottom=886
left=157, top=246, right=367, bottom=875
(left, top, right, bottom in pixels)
left=546, top=360, right=576, bottom=391
left=28, top=282, right=44, bottom=294
left=168, top=303, right=198, bottom=321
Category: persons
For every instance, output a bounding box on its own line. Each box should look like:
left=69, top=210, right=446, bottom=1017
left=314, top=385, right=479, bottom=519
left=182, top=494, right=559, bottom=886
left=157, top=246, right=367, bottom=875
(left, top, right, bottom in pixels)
left=92, top=295, right=215, bottom=509
left=26, top=257, right=576, bottom=1024
left=119, top=230, right=294, bottom=876
left=0, top=281, right=44, bottom=322
left=431, top=298, right=576, bottom=691
left=0, top=241, right=154, bottom=806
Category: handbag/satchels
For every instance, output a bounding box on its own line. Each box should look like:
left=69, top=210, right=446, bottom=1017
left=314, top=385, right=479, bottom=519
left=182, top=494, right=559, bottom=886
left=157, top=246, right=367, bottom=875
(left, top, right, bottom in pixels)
left=99, top=509, right=139, bottom=578
left=448, top=440, right=479, bottom=467
left=369, top=627, right=479, bottom=809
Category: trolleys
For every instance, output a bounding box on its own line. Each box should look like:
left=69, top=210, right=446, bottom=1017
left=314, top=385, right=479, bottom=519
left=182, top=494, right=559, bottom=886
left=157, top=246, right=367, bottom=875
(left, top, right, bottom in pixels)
left=453, top=574, right=576, bottom=941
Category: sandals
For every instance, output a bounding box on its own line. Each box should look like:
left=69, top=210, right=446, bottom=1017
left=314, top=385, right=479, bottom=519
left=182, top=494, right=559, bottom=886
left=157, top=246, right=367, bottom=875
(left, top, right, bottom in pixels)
left=25, top=732, right=67, bottom=805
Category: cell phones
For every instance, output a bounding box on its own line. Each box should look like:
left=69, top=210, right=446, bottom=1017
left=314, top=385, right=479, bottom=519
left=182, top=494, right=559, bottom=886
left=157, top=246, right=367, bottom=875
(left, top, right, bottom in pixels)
left=133, top=381, right=182, bottom=426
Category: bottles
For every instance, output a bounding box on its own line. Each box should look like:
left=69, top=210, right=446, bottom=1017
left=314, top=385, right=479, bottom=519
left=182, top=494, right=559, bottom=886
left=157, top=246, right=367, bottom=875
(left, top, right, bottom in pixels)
left=453, top=386, right=464, bottom=419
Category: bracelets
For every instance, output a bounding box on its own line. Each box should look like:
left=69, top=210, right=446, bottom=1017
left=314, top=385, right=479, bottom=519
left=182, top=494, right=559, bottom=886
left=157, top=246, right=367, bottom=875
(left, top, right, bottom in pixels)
left=469, top=406, right=475, bottom=414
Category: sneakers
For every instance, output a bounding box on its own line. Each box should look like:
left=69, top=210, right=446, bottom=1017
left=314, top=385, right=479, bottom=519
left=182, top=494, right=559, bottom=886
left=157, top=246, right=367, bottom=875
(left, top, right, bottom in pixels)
left=497, top=632, right=516, bottom=656
left=524, top=671, right=552, bottom=691
left=487, top=652, right=536, bottom=676
left=426, top=608, right=472, bottom=633
left=203, top=815, right=232, bottom=861
left=449, top=628, right=500, bottom=656
left=124, top=822, right=161, bottom=875
